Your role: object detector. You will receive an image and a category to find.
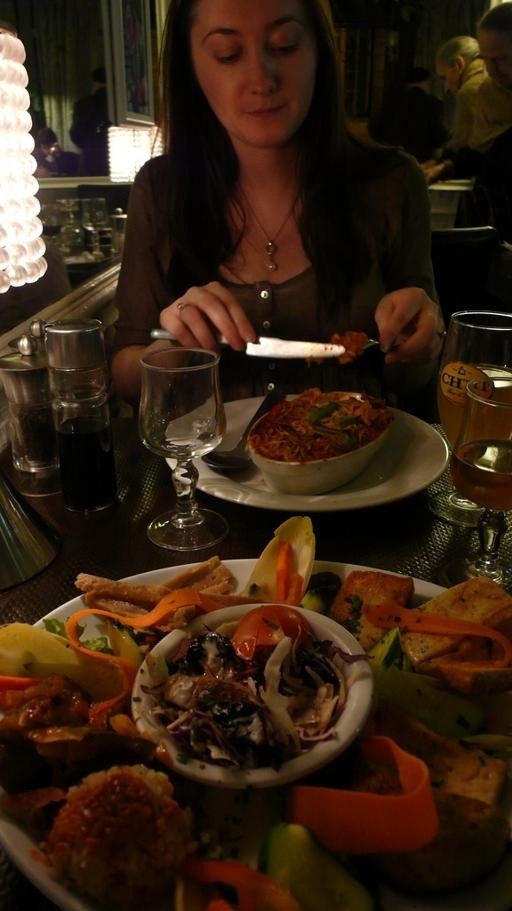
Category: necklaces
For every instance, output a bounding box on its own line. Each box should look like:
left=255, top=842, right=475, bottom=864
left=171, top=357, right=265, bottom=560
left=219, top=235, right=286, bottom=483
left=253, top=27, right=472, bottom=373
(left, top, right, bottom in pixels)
left=233, top=178, right=303, bottom=270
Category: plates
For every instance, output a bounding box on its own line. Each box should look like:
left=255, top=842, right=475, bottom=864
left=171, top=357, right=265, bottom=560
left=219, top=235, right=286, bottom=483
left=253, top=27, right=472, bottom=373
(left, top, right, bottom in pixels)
left=159, top=392, right=451, bottom=513
left=0, top=558, right=462, bottom=911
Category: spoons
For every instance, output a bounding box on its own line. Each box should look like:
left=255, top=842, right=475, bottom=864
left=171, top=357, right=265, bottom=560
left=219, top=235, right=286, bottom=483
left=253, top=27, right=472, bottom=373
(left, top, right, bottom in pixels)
left=201, top=379, right=288, bottom=471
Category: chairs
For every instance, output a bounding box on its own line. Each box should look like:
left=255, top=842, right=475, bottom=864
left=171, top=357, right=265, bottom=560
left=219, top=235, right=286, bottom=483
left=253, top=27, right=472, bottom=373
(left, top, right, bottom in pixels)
left=433, top=223, right=497, bottom=333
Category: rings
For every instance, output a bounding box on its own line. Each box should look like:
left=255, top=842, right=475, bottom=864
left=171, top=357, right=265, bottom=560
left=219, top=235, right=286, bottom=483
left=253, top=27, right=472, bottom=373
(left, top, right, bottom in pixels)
left=173, top=301, right=191, bottom=315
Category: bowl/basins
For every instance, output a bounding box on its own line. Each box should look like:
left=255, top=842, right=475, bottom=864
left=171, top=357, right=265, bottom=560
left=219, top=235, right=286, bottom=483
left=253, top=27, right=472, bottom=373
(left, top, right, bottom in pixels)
left=131, top=601, right=377, bottom=790
left=247, top=389, right=397, bottom=498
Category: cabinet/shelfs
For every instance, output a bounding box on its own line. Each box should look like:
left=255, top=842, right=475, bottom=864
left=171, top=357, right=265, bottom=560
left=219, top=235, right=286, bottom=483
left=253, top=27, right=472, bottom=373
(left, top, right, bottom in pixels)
left=37, top=178, right=134, bottom=275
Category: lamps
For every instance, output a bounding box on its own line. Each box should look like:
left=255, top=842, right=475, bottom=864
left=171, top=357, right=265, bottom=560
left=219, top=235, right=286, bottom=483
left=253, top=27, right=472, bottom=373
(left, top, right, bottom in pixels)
left=108, top=125, right=163, bottom=179
left=0, top=25, right=58, bottom=592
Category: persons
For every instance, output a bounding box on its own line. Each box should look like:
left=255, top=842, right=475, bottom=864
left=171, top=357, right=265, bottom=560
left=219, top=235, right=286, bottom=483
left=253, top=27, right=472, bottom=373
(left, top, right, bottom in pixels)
left=71, top=66, right=107, bottom=177
left=437, top=36, right=486, bottom=158
left=107, top=1, right=444, bottom=395
left=344, top=68, right=428, bottom=163
left=418, top=2, right=511, bottom=242
left=32, top=127, right=68, bottom=178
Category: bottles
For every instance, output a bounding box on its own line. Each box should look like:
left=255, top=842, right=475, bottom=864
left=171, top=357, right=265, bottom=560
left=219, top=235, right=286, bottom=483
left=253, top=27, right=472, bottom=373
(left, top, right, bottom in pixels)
left=43, top=321, right=115, bottom=515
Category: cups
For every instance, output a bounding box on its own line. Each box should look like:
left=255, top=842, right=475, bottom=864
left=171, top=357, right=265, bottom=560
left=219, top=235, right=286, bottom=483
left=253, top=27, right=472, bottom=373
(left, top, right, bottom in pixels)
left=447, top=377, right=512, bottom=585
left=138, top=344, right=230, bottom=552
left=428, top=308, right=512, bottom=529
left=81, top=197, right=110, bottom=262
left=54, top=197, right=81, bottom=264
